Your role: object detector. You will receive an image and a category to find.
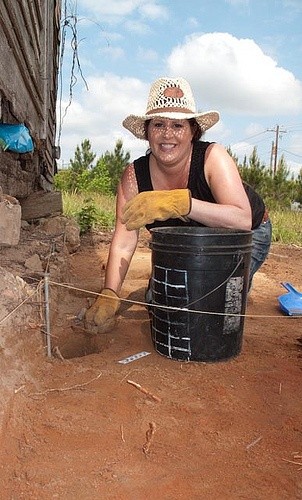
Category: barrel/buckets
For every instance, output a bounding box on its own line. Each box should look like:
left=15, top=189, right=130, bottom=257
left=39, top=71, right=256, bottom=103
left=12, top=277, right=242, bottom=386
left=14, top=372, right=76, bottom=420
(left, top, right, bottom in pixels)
left=146, top=226, right=254, bottom=363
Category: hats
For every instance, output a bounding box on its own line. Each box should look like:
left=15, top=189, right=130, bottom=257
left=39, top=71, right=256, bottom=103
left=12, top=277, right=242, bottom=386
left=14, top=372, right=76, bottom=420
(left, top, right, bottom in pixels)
left=122, top=78, right=220, bottom=140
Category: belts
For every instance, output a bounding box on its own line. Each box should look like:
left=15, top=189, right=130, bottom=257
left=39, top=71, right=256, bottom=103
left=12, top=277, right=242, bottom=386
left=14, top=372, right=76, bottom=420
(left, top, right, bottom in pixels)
left=262, top=210, right=270, bottom=222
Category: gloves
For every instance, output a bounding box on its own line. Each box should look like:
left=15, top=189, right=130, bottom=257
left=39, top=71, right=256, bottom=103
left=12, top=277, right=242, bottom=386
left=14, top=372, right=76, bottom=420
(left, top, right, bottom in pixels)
left=85, top=289, right=121, bottom=332
left=120, top=189, right=192, bottom=231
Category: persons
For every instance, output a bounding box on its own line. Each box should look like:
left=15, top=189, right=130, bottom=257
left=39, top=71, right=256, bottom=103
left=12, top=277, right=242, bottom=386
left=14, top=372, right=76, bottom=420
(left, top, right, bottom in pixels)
left=80, top=78, right=274, bottom=335
